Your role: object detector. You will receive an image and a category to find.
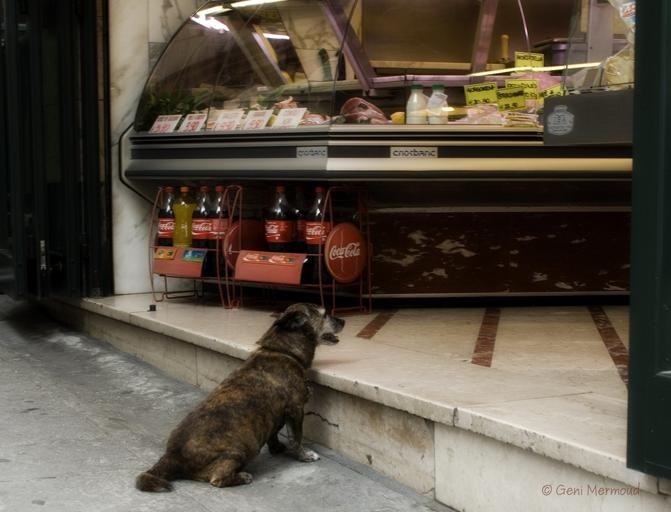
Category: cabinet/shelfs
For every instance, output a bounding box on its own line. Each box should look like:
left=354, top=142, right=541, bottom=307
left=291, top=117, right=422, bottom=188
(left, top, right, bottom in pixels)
left=147, top=179, right=373, bottom=317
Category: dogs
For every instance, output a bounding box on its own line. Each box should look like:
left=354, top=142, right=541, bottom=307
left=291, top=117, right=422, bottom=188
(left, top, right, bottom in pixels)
left=135, top=302, right=345, bottom=493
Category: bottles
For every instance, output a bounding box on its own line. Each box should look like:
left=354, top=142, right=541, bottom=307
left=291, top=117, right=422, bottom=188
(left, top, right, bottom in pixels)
left=304, top=186, right=332, bottom=284
left=155, top=186, right=174, bottom=245
left=209, top=186, right=229, bottom=275
left=405, top=83, right=425, bottom=124
left=172, top=186, right=197, bottom=246
left=265, top=186, right=292, bottom=252
left=288, top=186, right=311, bottom=282
left=429, top=84, right=449, bottom=124
left=192, top=186, right=213, bottom=274
